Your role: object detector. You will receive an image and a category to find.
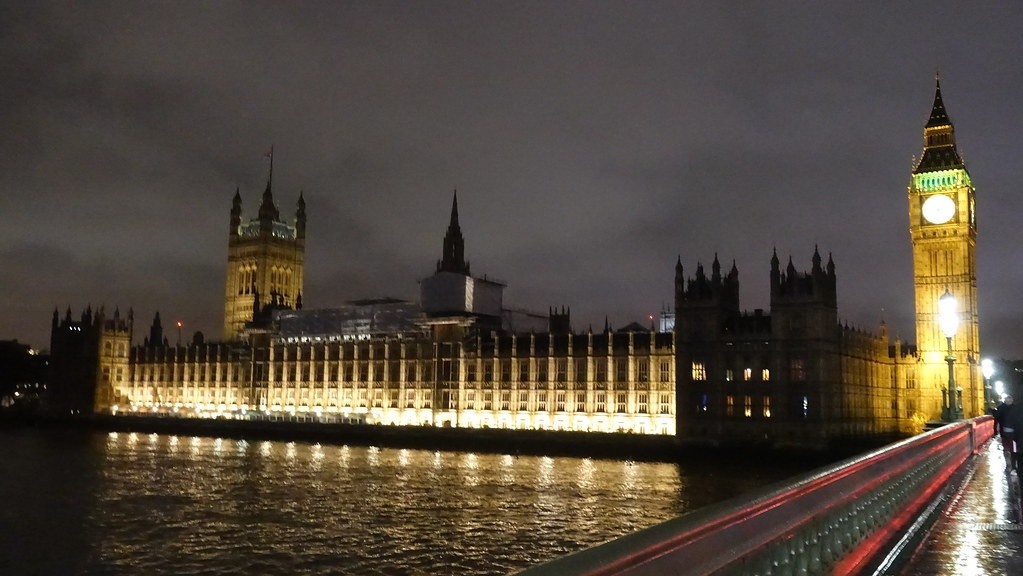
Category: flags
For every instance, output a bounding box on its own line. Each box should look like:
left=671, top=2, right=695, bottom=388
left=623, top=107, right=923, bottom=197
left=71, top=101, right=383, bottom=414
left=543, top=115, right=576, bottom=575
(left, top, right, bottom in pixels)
left=265, top=145, right=273, bottom=158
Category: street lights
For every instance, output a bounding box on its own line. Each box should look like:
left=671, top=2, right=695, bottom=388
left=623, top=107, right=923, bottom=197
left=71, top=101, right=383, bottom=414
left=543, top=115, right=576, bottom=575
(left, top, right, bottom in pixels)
left=938, top=289, right=965, bottom=422
left=981, top=359, right=1004, bottom=415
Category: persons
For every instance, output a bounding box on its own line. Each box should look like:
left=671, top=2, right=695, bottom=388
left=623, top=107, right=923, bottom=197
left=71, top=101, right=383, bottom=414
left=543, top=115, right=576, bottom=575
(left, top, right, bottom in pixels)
left=988, top=395, right=1017, bottom=473
